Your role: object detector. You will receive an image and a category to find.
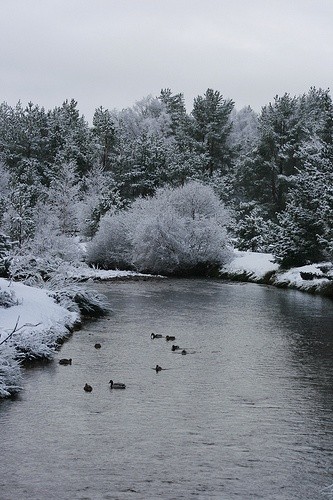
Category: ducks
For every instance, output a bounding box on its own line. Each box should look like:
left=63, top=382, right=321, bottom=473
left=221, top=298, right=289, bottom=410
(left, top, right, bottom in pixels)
left=182, top=349, right=186, bottom=355
left=155, top=333, right=163, bottom=338
left=156, top=364, right=162, bottom=370
left=166, top=335, right=175, bottom=341
left=171, top=344, right=176, bottom=352
left=172, top=346, right=179, bottom=349
left=83, top=382, right=92, bottom=390
left=108, top=379, right=126, bottom=388
left=151, top=332, right=155, bottom=339
left=58, top=358, right=72, bottom=365
left=94, top=344, right=101, bottom=349
